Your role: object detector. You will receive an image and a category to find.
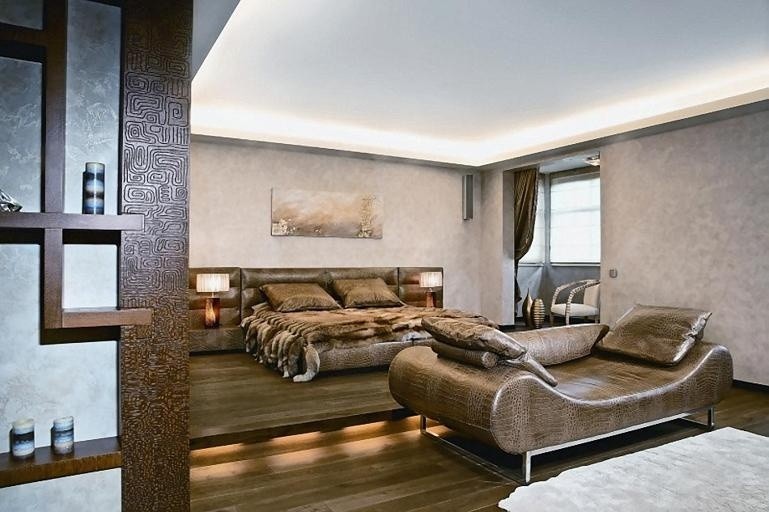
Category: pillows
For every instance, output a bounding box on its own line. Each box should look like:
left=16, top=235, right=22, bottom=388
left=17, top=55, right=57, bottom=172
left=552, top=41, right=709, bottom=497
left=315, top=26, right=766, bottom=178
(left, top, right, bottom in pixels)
left=596, top=301, right=714, bottom=365
left=420, top=312, right=558, bottom=382
left=259, top=281, right=339, bottom=312
left=333, top=280, right=403, bottom=307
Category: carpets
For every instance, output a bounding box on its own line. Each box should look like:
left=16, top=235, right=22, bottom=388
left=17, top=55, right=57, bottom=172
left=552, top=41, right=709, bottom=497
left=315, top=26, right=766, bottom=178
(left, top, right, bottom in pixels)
left=495, top=423, right=769, bottom=512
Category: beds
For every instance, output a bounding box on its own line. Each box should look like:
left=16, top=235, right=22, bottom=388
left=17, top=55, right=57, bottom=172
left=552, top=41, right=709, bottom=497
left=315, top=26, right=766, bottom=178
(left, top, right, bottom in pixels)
left=192, top=267, right=499, bottom=381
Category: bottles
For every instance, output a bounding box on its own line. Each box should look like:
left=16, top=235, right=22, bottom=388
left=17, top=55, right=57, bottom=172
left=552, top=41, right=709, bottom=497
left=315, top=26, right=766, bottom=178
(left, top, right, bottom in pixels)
left=51, top=417, right=74, bottom=456
left=522, top=287, right=545, bottom=328
left=9, top=419, right=35, bottom=458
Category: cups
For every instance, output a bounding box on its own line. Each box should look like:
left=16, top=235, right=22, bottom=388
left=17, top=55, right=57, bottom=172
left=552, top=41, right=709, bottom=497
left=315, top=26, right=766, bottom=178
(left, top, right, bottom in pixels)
left=82, top=160, right=106, bottom=214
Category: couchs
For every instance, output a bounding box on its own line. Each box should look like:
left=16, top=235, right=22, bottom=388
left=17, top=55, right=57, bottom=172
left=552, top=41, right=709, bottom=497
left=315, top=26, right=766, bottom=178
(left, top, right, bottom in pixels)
left=384, top=326, right=732, bottom=486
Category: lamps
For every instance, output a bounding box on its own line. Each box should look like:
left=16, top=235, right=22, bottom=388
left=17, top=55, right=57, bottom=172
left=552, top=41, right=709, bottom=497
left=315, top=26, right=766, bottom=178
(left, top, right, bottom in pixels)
left=418, top=270, right=443, bottom=307
left=192, top=272, right=231, bottom=329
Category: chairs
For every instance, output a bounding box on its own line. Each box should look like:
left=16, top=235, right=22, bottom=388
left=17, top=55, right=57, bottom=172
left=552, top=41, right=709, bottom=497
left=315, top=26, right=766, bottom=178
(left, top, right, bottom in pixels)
left=552, top=279, right=601, bottom=325
